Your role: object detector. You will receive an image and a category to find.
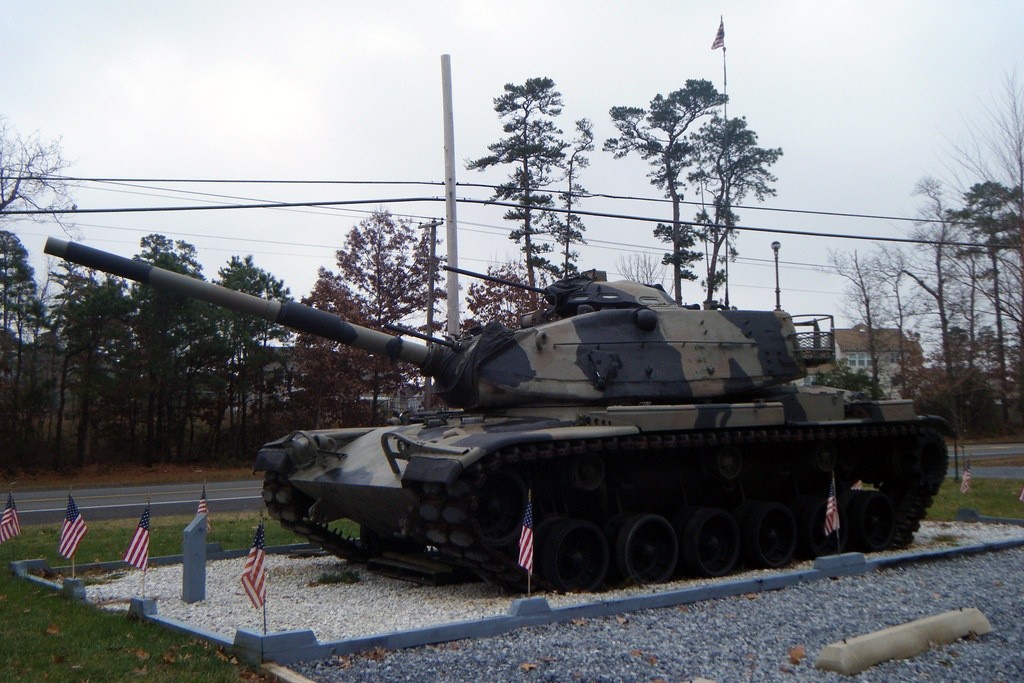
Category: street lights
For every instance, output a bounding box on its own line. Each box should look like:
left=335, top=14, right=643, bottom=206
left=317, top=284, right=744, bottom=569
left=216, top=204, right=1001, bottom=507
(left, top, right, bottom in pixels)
left=769, top=240, right=783, bottom=312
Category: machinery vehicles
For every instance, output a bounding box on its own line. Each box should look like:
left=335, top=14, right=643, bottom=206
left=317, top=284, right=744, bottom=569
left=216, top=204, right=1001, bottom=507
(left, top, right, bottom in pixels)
left=42, top=233, right=961, bottom=592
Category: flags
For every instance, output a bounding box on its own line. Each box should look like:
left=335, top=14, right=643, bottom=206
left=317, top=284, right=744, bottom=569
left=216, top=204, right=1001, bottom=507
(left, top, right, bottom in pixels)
left=1019, top=486, right=1024, bottom=502
left=518, top=500, right=532, bottom=576
left=0, top=492, right=22, bottom=544
left=59, top=495, right=88, bottom=560
left=197, top=485, right=210, bottom=534
left=240, top=516, right=266, bottom=609
left=123, top=503, right=149, bottom=572
left=825, top=481, right=840, bottom=535
left=960, top=462, right=971, bottom=493
